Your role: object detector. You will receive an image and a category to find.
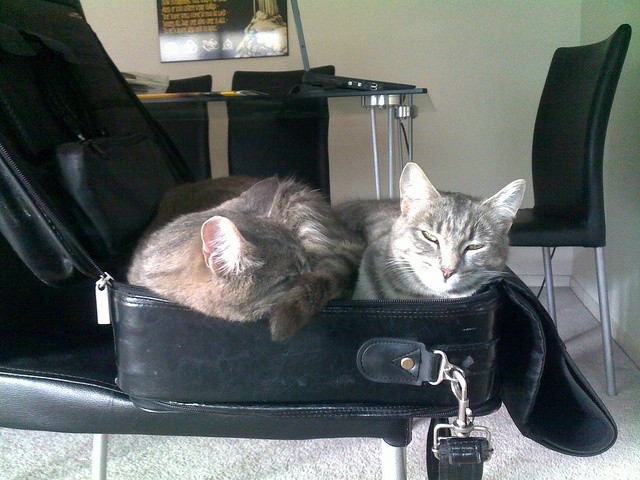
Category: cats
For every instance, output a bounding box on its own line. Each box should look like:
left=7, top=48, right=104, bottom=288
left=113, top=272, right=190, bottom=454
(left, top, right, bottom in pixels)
left=125, top=175, right=368, bottom=341
left=329, top=161, right=527, bottom=301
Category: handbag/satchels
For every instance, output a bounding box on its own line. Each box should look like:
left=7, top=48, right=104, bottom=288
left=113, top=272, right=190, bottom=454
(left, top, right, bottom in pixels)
left=0, top=0, right=618, bottom=476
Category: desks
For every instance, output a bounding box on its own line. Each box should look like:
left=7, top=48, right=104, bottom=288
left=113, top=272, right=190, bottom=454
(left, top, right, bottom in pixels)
left=131, top=86, right=429, bottom=200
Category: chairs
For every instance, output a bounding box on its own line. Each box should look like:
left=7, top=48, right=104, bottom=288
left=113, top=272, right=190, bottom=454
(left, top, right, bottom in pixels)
left=0, top=231, right=416, bottom=480
left=226, top=64, right=337, bottom=208
left=505, top=22, right=634, bottom=399
left=120, top=71, right=214, bottom=184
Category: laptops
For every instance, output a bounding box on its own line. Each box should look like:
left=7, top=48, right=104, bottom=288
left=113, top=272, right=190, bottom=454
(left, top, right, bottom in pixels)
left=290, top=0, right=417, bottom=96
